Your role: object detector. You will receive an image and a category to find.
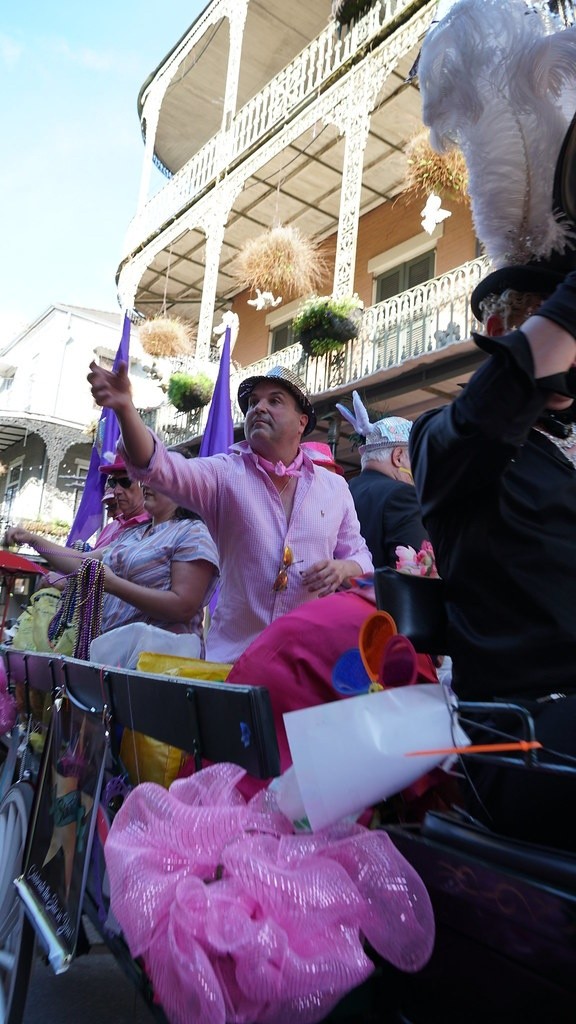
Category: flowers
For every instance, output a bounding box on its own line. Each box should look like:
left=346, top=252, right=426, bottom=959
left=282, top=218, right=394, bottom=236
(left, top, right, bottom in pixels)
left=394, top=539, right=442, bottom=580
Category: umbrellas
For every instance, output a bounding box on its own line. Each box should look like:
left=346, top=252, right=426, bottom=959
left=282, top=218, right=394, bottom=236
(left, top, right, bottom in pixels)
left=0, top=549, right=50, bottom=646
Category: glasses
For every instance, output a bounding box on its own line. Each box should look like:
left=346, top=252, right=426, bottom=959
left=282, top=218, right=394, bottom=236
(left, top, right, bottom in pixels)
left=272, top=546, right=293, bottom=592
left=107, top=478, right=131, bottom=489
left=106, top=503, right=117, bottom=511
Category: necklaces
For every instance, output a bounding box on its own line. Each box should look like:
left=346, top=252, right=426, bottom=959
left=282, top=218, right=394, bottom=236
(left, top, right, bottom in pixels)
left=530, top=422, right=576, bottom=471
left=47, top=557, right=107, bottom=662
left=278, top=475, right=294, bottom=497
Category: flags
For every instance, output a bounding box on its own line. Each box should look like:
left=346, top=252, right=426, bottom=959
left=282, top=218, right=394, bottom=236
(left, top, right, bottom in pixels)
left=64, top=318, right=131, bottom=549
left=196, top=328, right=234, bottom=458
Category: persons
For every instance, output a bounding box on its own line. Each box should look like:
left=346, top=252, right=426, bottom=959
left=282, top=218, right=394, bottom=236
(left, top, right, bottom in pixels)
left=0, top=479, right=221, bottom=672
left=345, top=416, right=433, bottom=572
left=35, top=453, right=154, bottom=592
left=407, top=248, right=576, bottom=852
left=85, top=360, right=375, bottom=664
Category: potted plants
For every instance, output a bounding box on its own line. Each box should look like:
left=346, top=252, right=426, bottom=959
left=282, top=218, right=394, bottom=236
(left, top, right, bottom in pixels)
left=167, top=370, right=214, bottom=413
left=292, top=293, right=365, bottom=358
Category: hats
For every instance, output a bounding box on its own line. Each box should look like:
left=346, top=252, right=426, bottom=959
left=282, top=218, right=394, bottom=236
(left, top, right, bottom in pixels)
left=299, top=442, right=344, bottom=474
left=99, top=454, right=129, bottom=475
left=101, top=475, right=116, bottom=502
left=358, top=415, right=413, bottom=454
left=470, top=262, right=568, bottom=326
left=237, top=366, right=317, bottom=439
